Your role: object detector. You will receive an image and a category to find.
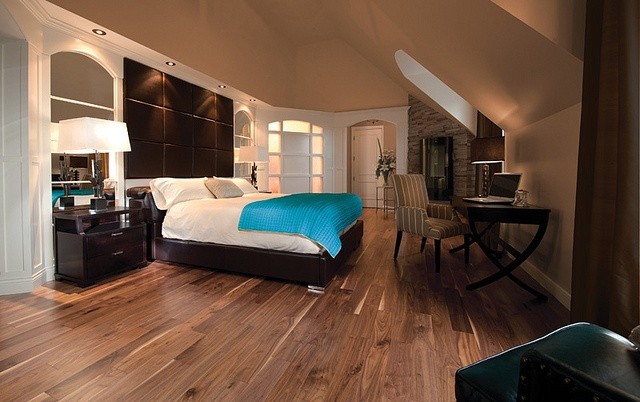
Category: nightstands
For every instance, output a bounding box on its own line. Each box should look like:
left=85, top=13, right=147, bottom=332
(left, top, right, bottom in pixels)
left=53, top=205, right=149, bottom=289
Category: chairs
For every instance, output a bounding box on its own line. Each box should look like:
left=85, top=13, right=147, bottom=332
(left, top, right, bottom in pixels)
left=393, top=172, right=471, bottom=273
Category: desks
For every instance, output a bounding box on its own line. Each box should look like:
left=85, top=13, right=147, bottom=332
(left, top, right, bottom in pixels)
left=447, top=194, right=552, bottom=303
left=375, top=184, right=397, bottom=216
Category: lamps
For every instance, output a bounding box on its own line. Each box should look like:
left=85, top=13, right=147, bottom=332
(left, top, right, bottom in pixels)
left=238, top=145, right=251, bottom=163
left=470, top=135, right=506, bottom=198
left=59, top=116, right=114, bottom=212
left=50, top=122, right=77, bottom=211
left=249, top=146, right=269, bottom=191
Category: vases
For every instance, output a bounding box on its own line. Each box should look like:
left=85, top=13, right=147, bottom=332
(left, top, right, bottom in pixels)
left=383, top=172, right=390, bottom=186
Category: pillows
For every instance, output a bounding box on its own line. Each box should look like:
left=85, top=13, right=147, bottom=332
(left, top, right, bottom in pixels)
left=154, top=177, right=216, bottom=208
left=228, top=177, right=257, bottom=193
left=205, top=178, right=244, bottom=199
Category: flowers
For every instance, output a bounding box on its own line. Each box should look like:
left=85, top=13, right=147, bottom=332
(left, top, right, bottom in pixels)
left=373, top=137, right=397, bottom=181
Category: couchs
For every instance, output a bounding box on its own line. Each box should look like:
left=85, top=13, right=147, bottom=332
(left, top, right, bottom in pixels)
left=453, top=319, right=640, bottom=402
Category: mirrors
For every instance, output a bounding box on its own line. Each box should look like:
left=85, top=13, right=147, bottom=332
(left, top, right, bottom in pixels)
left=234, top=109, right=253, bottom=178
left=47, top=51, right=119, bottom=214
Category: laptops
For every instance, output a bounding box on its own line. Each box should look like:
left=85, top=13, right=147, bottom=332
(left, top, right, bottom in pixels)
left=462, top=172, right=523, bottom=204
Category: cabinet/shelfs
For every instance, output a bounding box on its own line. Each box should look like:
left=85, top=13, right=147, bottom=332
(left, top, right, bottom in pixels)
left=420, top=136, right=455, bottom=200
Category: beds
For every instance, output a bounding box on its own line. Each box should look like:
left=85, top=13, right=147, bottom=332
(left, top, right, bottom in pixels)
left=126, top=178, right=364, bottom=294
